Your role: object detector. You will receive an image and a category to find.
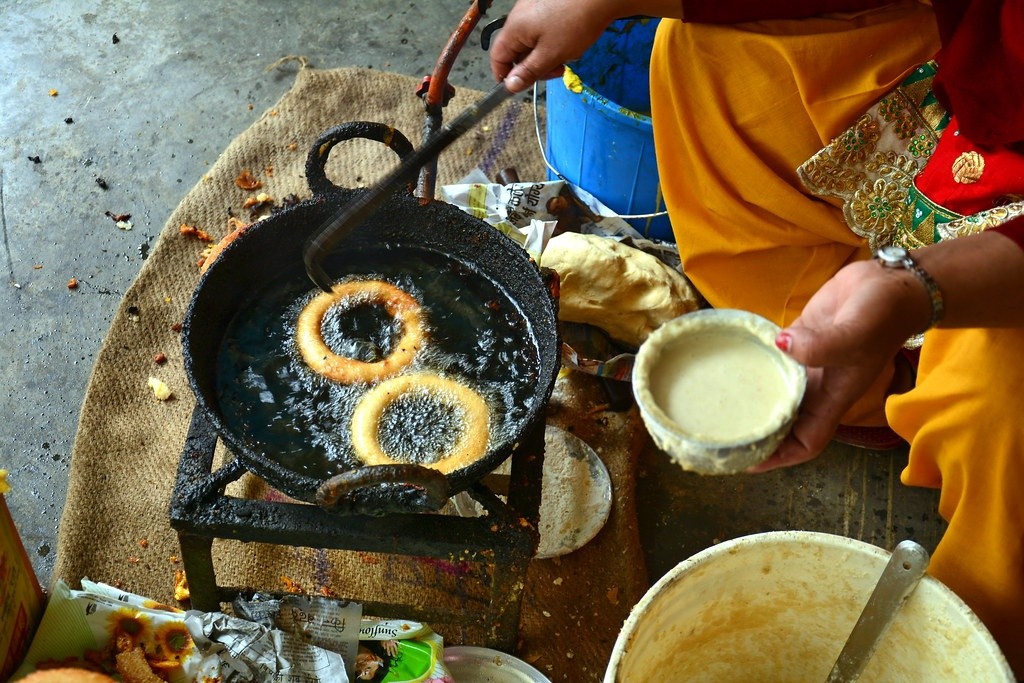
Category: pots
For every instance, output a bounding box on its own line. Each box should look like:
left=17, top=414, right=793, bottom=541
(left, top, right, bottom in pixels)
left=180, top=122, right=564, bottom=518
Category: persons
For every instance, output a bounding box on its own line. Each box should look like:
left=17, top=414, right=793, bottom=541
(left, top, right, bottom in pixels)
left=483, top=0, right=1024, bottom=683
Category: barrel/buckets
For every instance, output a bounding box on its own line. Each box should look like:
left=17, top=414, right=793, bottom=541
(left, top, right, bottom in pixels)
left=532, top=16, right=674, bottom=241
left=601, top=530, right=1016, bottom=682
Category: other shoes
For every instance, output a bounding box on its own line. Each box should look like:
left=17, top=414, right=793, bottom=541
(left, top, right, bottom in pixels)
left=832, top=346, right=919, bottom=451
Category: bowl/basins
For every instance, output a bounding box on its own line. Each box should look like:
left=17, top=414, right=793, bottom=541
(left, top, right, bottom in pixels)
left=632, top=308, right=809, bottom=477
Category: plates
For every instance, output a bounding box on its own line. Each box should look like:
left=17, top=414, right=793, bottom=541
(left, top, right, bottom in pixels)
left=443, top=647, right=552, bottom=682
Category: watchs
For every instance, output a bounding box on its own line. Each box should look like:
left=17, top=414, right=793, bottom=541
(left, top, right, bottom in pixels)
left=872, top=245, right=944, bottom=334
